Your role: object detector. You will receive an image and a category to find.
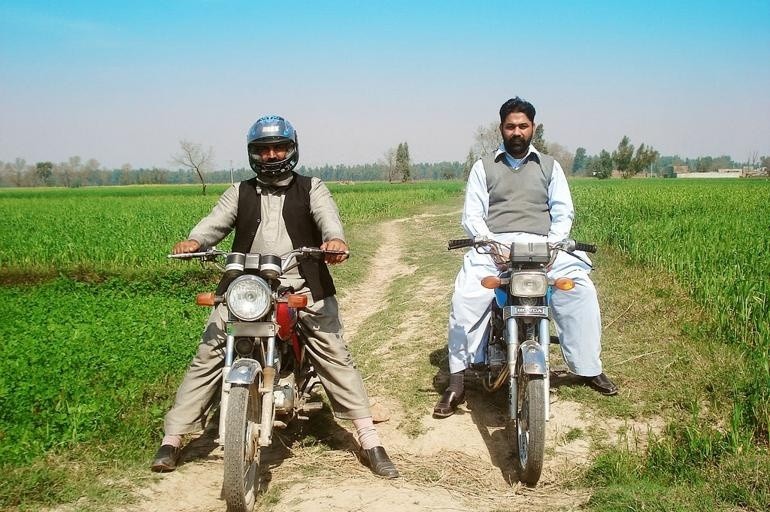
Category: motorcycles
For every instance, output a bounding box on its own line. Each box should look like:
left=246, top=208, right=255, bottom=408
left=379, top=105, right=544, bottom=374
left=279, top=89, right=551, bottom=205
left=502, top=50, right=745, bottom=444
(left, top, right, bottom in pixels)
left=447, top=235, right=597, bottom=486
left=167, top=246, right=349, bottom=512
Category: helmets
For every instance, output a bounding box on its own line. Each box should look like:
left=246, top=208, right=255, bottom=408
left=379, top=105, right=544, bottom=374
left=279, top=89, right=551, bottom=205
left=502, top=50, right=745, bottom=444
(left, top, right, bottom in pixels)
left=247, top=115, right=299, bottom=177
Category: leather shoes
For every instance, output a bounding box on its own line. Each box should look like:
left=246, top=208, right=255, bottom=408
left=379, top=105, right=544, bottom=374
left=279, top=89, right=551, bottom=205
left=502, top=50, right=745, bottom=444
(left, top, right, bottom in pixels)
left=359, top=445, right=399, bottom=478
left=583, top=373, right=618, bottom=396
left=434, top=385, right=466, bottom=417
left=150, top=445, right=183, bottom=470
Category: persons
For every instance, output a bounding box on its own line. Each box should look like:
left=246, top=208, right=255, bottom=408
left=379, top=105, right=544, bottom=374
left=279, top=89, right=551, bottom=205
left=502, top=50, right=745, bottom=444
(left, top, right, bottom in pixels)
left=151, top=115, right=400, bottom=482
left=431, top=93, right=619, bottom=419
